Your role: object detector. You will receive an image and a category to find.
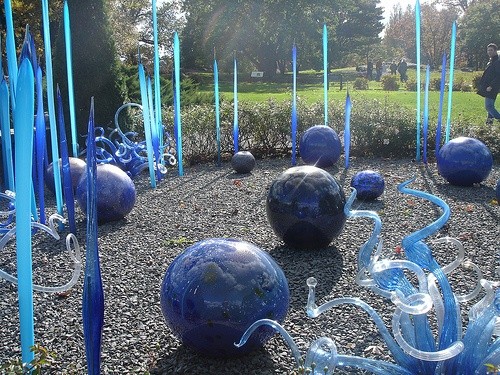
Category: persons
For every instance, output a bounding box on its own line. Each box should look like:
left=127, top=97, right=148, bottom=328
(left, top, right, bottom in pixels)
left=382, top=62, right=389, bottom=76
left=390, top=60, right=397, bottom=75
left=398, top=58, right=408, bottom=82
left=476, top=44, right=500, bottom=126
left=367, top=58, right=373, bottom=78
left=375, top=57, right=382, bottom=82
